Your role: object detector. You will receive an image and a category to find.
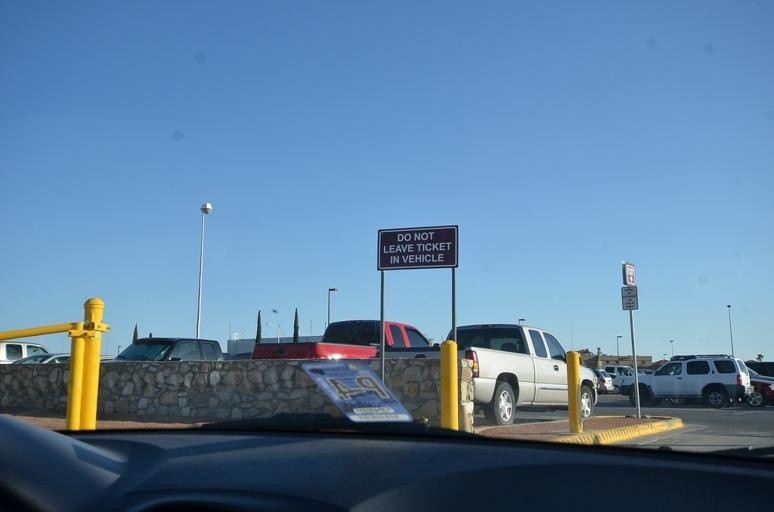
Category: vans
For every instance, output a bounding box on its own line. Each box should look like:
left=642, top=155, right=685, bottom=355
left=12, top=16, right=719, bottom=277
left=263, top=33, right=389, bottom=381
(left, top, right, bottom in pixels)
left=0, top=341, right=49, bottom=364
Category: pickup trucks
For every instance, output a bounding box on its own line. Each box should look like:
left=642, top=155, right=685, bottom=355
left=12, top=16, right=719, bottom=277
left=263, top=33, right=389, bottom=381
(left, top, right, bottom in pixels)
left=252, top=320, right=440, bottom=359
left=441, top=324, right=598, bottom=427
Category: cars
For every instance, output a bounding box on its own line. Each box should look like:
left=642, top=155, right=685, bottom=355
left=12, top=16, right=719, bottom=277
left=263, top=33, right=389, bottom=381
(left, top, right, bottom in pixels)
left=668, top=365, right=774, bottom=408
left=595, top=365, right=654, bottom=394
left=222, top=351, right=252, bottom=360
left=10, top=353, right=71, bottom=363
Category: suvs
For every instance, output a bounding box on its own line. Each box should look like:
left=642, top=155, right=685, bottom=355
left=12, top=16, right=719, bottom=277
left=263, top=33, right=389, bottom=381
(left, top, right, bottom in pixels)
left=618, top=354, right=754, bottom=407
left=100, top=337, right=224, bottom=361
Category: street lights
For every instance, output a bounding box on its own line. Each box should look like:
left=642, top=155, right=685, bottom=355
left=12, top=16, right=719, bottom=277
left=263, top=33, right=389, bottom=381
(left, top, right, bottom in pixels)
left=264, top=321, right=280, bottom=344
left=669, top=339, right=674, bottom=357
left=726, top=304, right=734, bottom=356
left=195, top=200, right=212, bottom=338
left=518, top=318, right=525, bottom=324
left=326, top=287, right=337, bottom=325
left=616, top=335, right=622, bottom=357
